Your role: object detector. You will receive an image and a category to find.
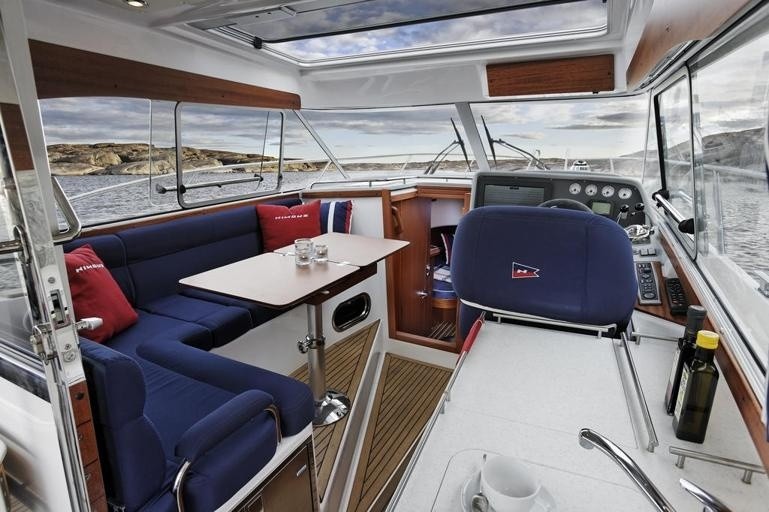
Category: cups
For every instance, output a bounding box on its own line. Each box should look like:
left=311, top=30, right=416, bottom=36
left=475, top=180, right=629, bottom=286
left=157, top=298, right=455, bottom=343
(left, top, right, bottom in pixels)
left=311, top=243, right=329, bottom=263
left=480, top=453, right=542, bottom=511
left=294, top=238, right=313, bottom=266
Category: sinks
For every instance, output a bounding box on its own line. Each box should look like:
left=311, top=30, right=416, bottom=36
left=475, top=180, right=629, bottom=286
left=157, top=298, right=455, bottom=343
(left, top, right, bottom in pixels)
left=430, top=448, right=656, bottom=511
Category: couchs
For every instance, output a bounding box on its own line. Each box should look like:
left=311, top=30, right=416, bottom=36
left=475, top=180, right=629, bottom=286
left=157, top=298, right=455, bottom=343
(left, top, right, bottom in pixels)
left=57, top=198, right=320, bottom=512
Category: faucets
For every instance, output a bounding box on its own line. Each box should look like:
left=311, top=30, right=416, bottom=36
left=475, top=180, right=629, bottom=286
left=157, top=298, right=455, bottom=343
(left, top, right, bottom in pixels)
left=579, top=427, right=733, bottom=512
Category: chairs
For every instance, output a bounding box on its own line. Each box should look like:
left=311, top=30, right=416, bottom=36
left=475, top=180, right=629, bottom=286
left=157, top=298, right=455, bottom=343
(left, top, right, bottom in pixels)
left=450, top=205, right=638, bottom=342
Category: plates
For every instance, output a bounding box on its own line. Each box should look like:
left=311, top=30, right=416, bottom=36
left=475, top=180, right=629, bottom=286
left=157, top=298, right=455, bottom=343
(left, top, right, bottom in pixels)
left=462, top=471, right=558, bottom=511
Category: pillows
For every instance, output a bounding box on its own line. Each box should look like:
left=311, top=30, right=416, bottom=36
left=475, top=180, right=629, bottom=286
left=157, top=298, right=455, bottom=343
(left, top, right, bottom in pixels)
left=64, top=245, right=138, bottom=342
left=256, top=199, right=322, bottom=252
left=321, top=199, right=354, bottom=234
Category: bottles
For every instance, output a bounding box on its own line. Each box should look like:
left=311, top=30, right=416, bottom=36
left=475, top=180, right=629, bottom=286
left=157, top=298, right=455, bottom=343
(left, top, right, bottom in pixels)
left=672, top=330, right=721, bottom=444
left=664, top=304, right=707, bottom=417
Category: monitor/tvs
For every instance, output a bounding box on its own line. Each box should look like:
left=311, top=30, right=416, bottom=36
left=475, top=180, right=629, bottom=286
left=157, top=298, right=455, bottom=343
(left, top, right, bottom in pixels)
left=475, top=176, right=554, bottom=209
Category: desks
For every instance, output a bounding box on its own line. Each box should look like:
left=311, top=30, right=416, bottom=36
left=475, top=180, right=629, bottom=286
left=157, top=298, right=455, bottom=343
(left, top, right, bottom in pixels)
left=179, top=232, right=410, bottom=427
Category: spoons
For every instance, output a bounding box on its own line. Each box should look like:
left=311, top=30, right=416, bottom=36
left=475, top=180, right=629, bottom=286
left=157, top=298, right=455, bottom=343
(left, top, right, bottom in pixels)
left=470, top=455, right=489, bottom=512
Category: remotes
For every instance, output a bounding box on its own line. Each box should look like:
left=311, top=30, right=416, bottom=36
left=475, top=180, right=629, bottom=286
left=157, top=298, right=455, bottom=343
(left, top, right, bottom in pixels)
left=664, top=278, right=688, bottom=316
